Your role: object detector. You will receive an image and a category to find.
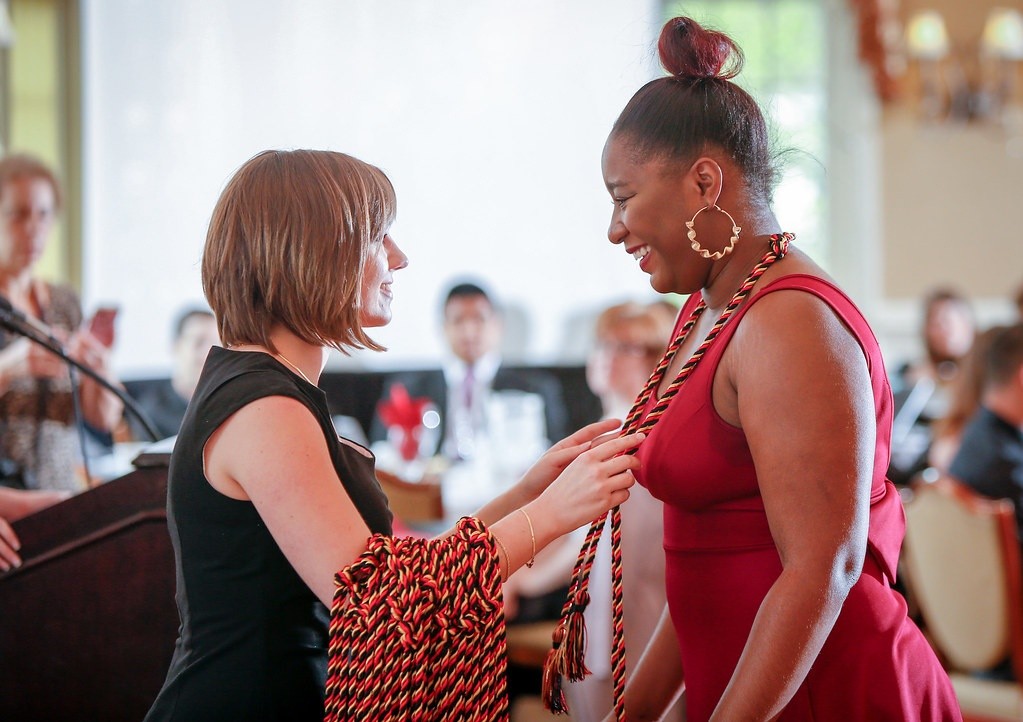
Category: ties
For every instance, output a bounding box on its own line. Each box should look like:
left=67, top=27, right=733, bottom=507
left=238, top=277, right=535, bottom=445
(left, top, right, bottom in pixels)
left=463, top=362, right=475, bottom=410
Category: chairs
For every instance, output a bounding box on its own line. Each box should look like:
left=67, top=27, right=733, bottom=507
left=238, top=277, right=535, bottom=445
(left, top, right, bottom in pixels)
left=373, top=468, right=444, bottom=524
left=891, top=469, right=1023, bottom=722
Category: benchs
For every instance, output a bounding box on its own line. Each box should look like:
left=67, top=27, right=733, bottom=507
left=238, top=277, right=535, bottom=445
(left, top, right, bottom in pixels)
left=123, top=369, right=600, bottom=450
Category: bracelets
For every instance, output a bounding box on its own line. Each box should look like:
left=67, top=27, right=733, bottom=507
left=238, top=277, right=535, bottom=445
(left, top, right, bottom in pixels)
left=489, top=531, right=512, bottom=587
left=519, top=509, right=536, bottom=568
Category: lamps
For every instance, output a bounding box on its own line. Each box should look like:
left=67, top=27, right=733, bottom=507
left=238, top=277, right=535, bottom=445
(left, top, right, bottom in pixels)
left=903, top=0, right=1023, bottom=123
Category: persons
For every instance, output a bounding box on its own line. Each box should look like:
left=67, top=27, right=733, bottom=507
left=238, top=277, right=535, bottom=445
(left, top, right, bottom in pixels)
left=600, top=18, right=963, bottom=722
left=145, top=146, right=645, bottom=722
left=0, top=159, right=1023, bottom=722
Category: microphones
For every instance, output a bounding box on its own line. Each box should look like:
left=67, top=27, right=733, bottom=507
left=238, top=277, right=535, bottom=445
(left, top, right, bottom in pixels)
left=0, top=296, right=57, bottom=342
